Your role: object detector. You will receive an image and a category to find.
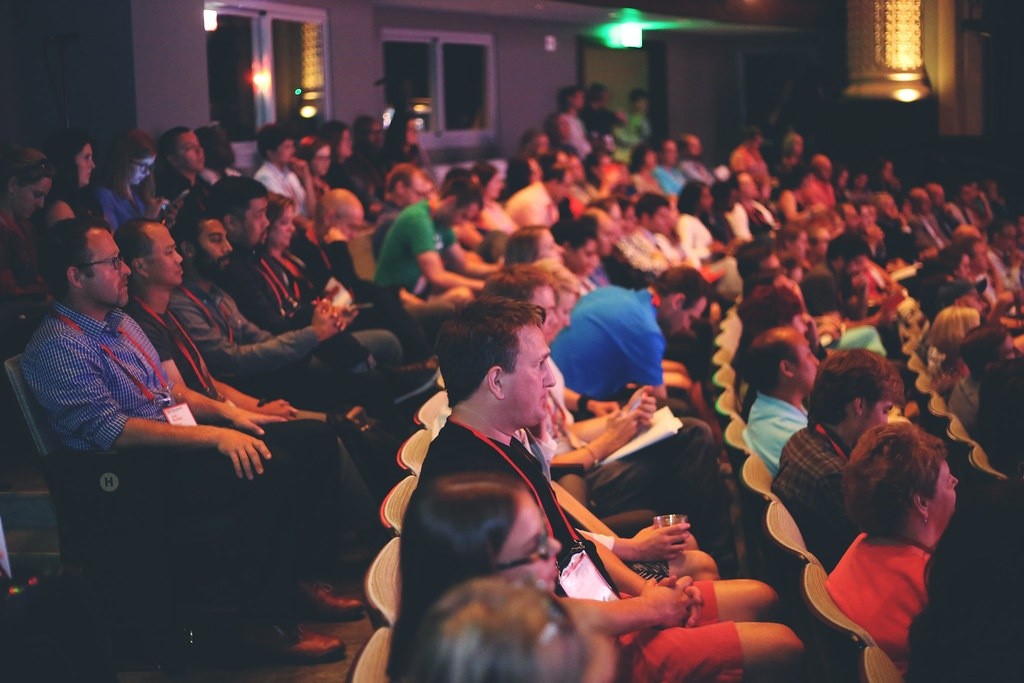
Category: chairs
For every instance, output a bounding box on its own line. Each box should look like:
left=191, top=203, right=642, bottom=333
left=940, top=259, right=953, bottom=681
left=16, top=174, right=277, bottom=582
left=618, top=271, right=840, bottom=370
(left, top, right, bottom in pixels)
left=347, top=368, right=451, bottom=683
left=711, top=292, right=1008, bottom=683
left=0, top=353, right=244, bottom=683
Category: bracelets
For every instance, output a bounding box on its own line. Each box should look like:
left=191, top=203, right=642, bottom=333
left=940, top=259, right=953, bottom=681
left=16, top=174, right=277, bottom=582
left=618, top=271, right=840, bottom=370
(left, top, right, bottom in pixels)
left=577, top=394, right=592, bottom=411
left=582, top=445, right=600, bottom=468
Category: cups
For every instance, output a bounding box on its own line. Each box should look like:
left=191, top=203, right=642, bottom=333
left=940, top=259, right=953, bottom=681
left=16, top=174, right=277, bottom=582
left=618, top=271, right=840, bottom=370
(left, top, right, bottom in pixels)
left=653, top=514, right=688, bottom=545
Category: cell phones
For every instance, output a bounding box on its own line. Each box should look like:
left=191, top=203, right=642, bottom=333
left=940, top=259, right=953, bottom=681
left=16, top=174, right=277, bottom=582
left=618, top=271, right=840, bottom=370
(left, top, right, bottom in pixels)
left=164, top=188, right=191, bottom=212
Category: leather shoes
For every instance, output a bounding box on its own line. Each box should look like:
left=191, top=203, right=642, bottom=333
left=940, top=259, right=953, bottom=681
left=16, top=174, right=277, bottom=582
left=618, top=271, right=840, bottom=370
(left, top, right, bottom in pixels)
left=286, top=580, right=367, bottom=618
left=240, top=619, right=347, bottom=664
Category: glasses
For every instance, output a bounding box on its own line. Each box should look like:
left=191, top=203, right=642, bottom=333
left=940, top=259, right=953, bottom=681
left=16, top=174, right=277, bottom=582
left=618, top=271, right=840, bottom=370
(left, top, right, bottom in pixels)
left=16, top=161, right=49, bottom=174
left=487, top=523, right=551, bottom=575
left=131, top=160, right=155, bottom=173
left=75, top=254, right=125, bottom=269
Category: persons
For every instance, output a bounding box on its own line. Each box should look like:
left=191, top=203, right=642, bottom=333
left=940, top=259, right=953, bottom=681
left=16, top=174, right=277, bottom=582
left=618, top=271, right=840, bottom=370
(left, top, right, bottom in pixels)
left=0, top=110, right=1024, bottom=683
left=581, top=83, right=624, bottom=141
left=552, top=86, right=591, bottom=160
left=612, top=89, right=655, bottom=165
